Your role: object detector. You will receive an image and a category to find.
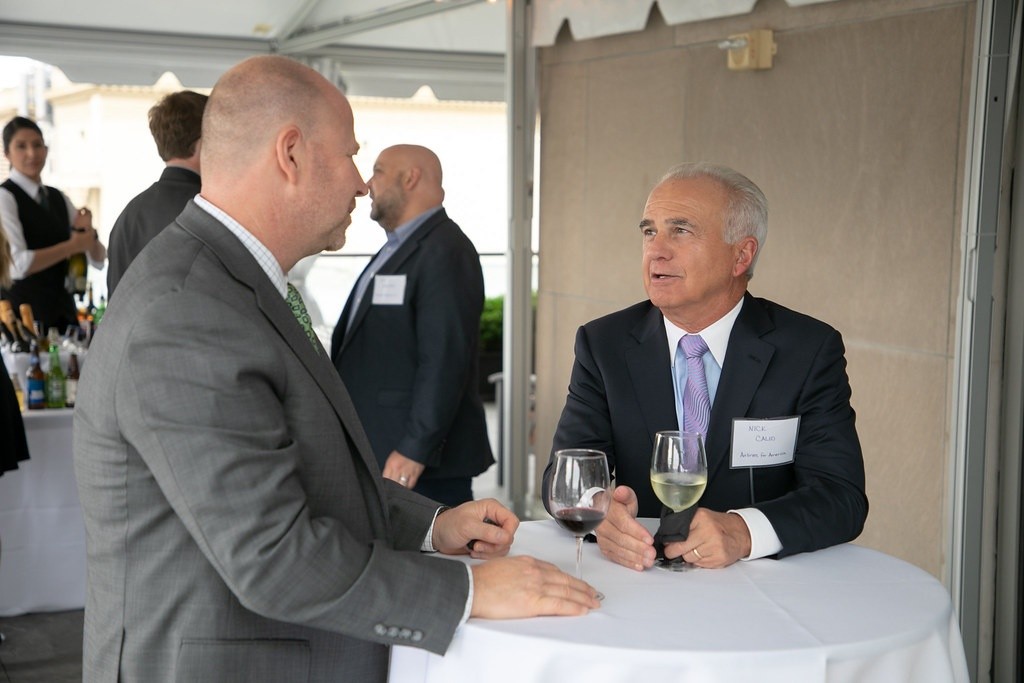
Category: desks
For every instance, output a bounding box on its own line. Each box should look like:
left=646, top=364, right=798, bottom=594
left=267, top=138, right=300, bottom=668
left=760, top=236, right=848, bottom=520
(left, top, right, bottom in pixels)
left=385, top=517, right=971, bottom=683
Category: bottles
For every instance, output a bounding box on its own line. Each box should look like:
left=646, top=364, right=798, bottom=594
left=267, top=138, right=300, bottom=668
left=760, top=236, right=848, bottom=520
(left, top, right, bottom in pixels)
left=45, top=345, right=67, bottom=408
left=65, top=355, right=79, bottom=406
left=68, top=226, right=87, bottom=296
left=0, top=283, right=106, bottom=352
left=25, top=345, right=46, bottom=408
left=10, top=372, right=23, bottom=411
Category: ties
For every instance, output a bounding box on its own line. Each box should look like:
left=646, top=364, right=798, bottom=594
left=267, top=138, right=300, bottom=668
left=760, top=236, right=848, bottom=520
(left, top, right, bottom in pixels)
left=283, top=282, right=319, bottom=355
left=680, top=334, right=711, bottom=474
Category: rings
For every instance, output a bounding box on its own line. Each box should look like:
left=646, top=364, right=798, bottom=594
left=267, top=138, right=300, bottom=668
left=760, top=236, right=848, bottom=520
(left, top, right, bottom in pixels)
left=400, top=477, right=408, bottom=482
left=693, top=548, right=703, bottom=559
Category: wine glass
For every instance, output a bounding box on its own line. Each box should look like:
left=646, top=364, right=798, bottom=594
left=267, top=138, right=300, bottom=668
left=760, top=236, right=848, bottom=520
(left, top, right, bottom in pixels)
left=549, top=449, right=610, bottom=601
left=649, top=430, right=707, bottom=573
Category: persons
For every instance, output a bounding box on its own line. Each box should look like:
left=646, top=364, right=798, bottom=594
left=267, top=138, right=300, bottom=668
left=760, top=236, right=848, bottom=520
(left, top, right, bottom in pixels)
left=73, top=55, right=602, bottom=683
left=541, top=162, right=869, bottom=571
left=331, top=144, right=497, bottom=509
left=106, top=91, right=208, bottom=306
left=0, top=116, right=106, bottom=341
